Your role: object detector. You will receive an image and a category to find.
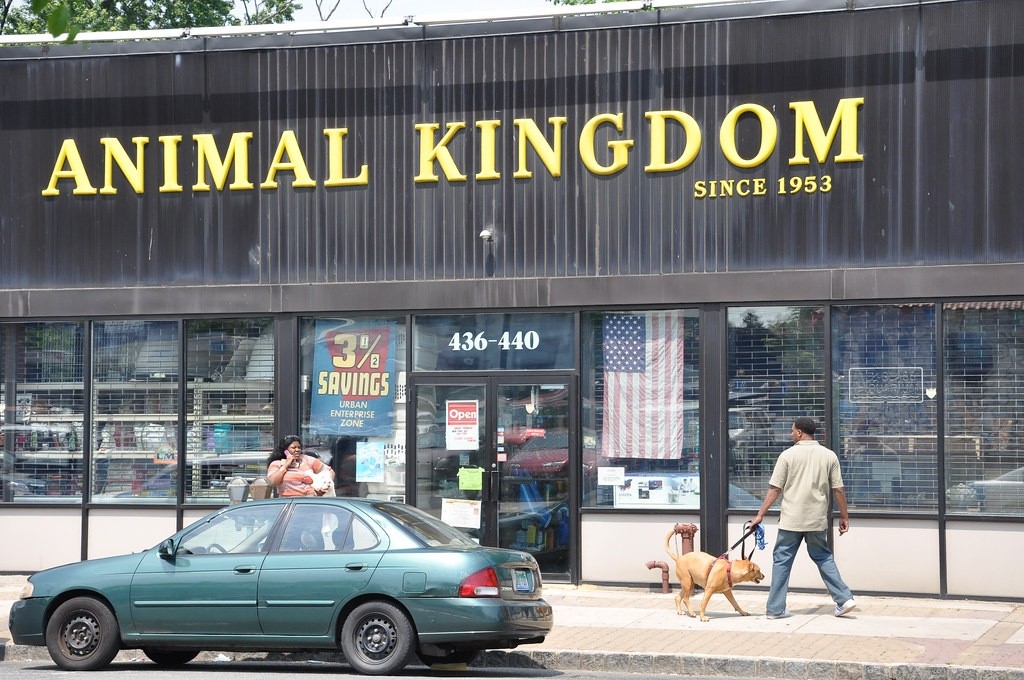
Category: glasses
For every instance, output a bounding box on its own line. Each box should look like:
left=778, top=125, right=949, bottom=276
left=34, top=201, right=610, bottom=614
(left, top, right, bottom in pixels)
left=283, top=435, right=301, bottom=441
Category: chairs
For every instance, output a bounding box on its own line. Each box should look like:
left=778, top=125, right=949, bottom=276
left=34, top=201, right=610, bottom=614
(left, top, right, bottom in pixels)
left=332, top=524, right=354, bottom=549
left=301, top=524, right=325, bottom=550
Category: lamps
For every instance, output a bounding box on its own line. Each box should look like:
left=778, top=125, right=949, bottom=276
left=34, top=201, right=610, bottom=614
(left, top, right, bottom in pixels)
left=478, top=229, right=494, bottom=242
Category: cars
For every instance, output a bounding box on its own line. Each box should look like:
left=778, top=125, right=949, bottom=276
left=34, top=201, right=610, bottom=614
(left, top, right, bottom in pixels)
left=106, top=407, right=1024, bottom=562
left=10, top=496, right=552, bottom=676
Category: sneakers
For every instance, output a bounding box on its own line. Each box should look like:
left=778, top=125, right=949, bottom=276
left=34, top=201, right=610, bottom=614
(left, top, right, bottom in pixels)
left=766, top=609, right=791, bottom=620
left=835, top=599, right=856, bottom=617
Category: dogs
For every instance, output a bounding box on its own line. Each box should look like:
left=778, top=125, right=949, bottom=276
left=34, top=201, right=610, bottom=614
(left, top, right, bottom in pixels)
left=305, top=468, right=337, bottom=496
left=664, top=530, right=765, bottom=621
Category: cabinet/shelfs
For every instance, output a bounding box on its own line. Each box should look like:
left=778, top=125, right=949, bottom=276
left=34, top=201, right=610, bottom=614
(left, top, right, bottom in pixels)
left=0, top=381, right=274, bottom=505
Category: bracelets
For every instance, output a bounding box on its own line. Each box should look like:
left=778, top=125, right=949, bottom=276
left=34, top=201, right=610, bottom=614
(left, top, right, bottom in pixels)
left=280, top=466, right=287, bottom=473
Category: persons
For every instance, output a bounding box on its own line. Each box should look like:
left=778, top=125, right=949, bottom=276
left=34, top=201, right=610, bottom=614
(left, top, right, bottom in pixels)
left=331, top=436, right=363, bottom=485
left=267, top=436, right=336, bottom=549
left=749, top=417, right=857, bottom=619
left=841, top=420, right=900, bottom=504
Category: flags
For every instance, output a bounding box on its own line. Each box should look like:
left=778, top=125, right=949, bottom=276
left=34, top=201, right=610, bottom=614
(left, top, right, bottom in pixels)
left=603, top=311, right=683, bottom=459
